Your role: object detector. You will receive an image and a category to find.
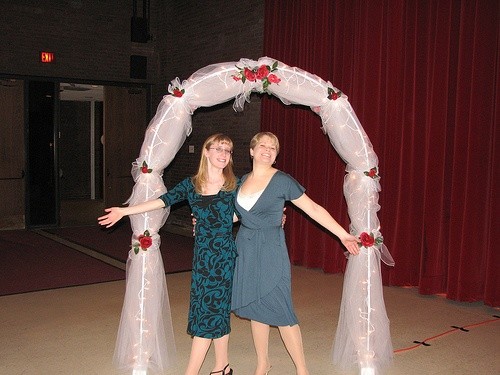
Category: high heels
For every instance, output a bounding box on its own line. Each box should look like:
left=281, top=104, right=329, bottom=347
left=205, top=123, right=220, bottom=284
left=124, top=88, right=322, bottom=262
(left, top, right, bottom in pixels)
left=209, top=363, right=233, bottom=375
left=263, top=360, right=272, bottom=375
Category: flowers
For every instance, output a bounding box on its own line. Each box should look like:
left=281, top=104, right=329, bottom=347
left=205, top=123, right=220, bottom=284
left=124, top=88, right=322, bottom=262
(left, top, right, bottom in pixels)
left=242, top=65, right=280, bottom=86
left=369, top=169, right=376, bottom=177
left=331, top=92, right=338, bottom=101
left=173, top=89, right=181, bottom=96
left=137, top=234, right=152, bottom=252
left=141, top=166, right=147, bottom=174
left=359, top=232, right=374, bottom=248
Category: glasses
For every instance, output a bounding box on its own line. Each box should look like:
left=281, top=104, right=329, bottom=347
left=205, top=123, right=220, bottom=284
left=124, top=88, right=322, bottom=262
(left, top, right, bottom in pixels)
left=209, top=146, right=233, bottom=154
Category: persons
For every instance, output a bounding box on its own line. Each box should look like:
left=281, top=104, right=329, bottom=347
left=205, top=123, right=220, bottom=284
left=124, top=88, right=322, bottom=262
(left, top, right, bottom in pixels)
left=190, top=129, right=362, bottom=375
left=97, top=135, right=287, bottom=375
